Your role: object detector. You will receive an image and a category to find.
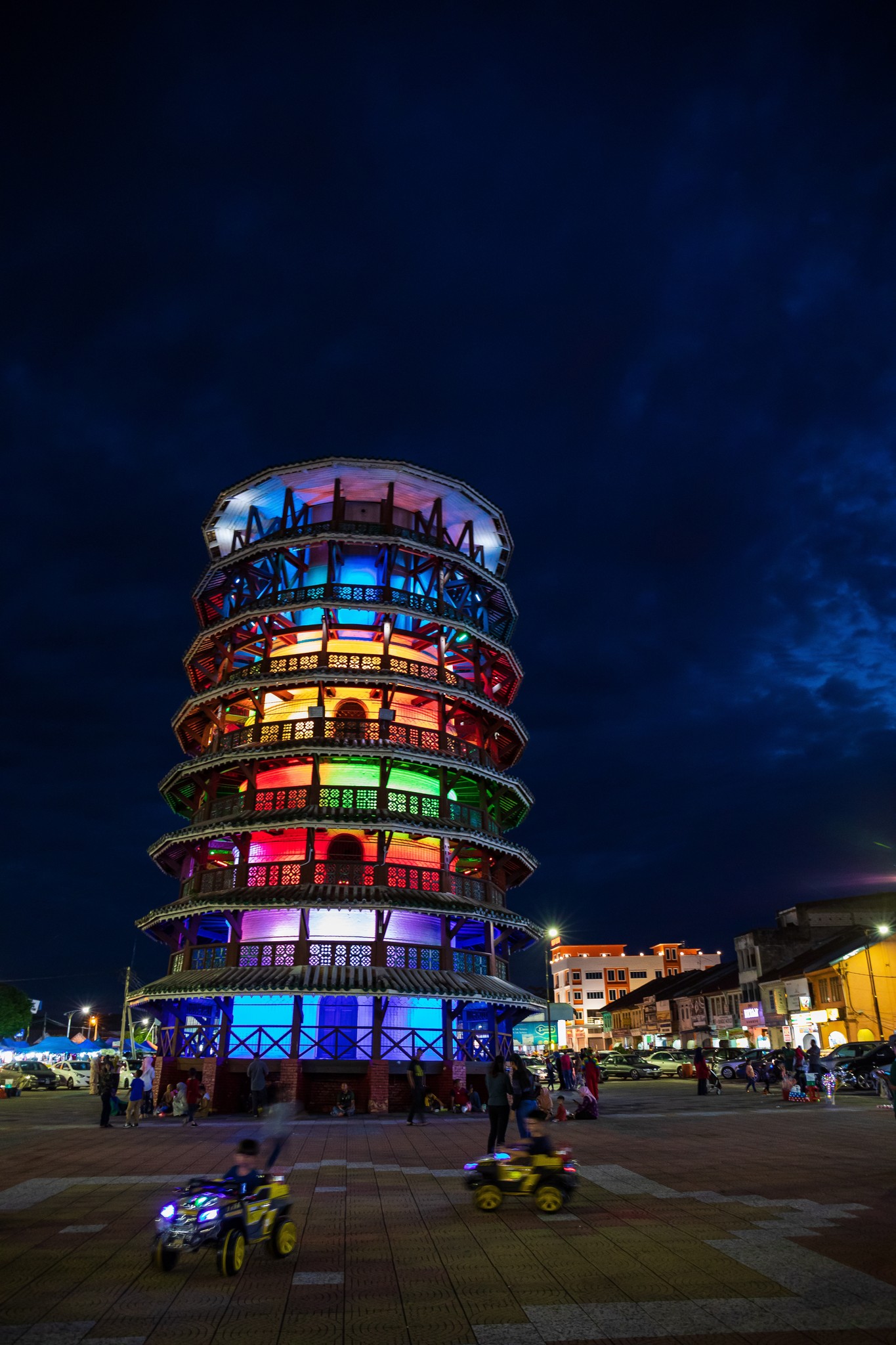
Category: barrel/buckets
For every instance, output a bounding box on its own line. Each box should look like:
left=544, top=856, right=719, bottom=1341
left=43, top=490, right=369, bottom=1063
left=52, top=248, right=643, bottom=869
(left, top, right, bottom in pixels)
left=12, top=1087, right=16, bottom=1097
left=5, top=1088, right=11, bottom=1098
left=16, top=1089, right=21, bottom=1097
left=806, top=1073, right=815, bottom=1086
left=5, top=1079, right=13, bottom=1088
left=682, top=1063, right=693, bottom=1078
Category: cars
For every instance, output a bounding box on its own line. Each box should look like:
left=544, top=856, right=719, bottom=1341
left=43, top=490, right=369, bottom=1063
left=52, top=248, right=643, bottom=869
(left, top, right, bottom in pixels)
left=464, top=1141, right=582, bottom=1213
left=820, top=1041, right=896, bottom=1092
left=680, top=1048, right=811, bottom=1083
left=516, top=1053, right=558, bottom=1084
left=593, top=1051, right=663, bottom=1080
left=156, top=1178, right=299, bottom=1276
left=632, top=1046, right=698, bottom=1077
left=548, top=1052, right=581, bottom=1065
left=0, top=1061, right=60, bottom=1090
left=118, top=1060, right=144, bottom=1089
left=47, top=1061, right=93, bottom=1091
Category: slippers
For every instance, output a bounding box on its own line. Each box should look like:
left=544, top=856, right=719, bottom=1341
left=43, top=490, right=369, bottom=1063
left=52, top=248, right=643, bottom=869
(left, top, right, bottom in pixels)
left=406, top=1121, right=418, bottom=1126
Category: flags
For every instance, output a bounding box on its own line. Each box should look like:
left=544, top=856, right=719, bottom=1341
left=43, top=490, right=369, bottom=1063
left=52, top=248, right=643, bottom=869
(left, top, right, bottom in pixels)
left=30, top=999, right=42, bottom=1014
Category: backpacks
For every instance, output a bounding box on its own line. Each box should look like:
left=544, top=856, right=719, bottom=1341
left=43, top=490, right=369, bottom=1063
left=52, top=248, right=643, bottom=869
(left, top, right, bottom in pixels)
left=523, top=1074, right=542, bottom=1100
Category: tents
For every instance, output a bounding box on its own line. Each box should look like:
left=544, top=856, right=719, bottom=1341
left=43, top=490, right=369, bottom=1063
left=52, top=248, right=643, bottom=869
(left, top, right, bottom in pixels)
left=0, top=1033, right=159, bottom=1061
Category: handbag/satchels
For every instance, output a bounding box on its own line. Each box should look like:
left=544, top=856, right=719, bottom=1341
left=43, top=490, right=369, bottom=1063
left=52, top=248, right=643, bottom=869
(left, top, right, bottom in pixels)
left=480, top=1104, right=487, bottom=1112
left=466, top=1101, right=472, bottom=1112
left=461, top=1106, right=468, bottom=1113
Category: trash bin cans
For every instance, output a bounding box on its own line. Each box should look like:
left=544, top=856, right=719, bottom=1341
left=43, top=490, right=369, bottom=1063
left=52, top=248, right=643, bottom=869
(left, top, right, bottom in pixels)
left=682, top=1063, right=693, bottom=1077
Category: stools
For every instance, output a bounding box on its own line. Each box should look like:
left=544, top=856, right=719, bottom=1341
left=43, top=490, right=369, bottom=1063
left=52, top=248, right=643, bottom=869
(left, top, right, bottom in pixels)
left=805, top=1086, right=820, bottom=1103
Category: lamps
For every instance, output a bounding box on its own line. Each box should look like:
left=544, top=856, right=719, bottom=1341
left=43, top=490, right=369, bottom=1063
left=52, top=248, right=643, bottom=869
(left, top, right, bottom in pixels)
left=308, top=706, right=325, bottom=718
left=378, top=708, right=395, bottom=721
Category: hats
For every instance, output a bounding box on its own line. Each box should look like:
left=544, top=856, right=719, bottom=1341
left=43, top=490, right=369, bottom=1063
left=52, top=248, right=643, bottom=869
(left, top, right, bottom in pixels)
left=235, top=1138, right=260, bottom=1156
left=586, top=1049, right=594, bottom=1056
left=886, top=1034, right=896, bottom=1047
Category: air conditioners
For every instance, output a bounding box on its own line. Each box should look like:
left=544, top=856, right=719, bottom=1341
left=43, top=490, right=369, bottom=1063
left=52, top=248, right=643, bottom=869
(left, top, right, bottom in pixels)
left=594, top=1019, right=600, bottom=1025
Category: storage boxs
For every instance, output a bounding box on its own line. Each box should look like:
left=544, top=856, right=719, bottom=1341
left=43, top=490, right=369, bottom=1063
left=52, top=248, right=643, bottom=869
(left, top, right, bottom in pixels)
left=781, top=1088, right=790, bottom=1101
left=4, top=1087, right=21, bottom=1098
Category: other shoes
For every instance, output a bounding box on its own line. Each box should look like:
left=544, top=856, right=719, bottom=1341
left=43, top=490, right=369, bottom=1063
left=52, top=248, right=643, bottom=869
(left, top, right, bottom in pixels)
left=754, top=1090, right=760, bottom=1093
left=122, top=1123, right=131, bottom=1128
left=191, top=1122, right=198, bottom=1127
left=182, top=1118, right=187, bottom=1127
left=131, top=1123, right=139, bottom=1127
left=546, top=1087, right=550, bottom=1091
left=100, top=1124, right=114, bottom=1128
left=743, top=1088, right=750, bottom=1093
left=551, top=1089, right=555, bottom=1091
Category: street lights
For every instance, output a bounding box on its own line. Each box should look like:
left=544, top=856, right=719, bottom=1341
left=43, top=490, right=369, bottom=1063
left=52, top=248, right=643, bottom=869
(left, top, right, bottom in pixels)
left=63, top=1007, right=89, bottom=1038
left=89, top=1018, right=98, bottom=1040
left=128, top=1019, right=148, bottom=1060
left=543, top=927, right=560, bottom=1052
left=863, top=926, right=889, bottom=1042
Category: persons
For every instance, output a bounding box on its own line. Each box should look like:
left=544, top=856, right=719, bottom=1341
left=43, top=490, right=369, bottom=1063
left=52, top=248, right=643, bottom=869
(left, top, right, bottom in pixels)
left=534, top=1047, right=602, bottom=1122
left=41, top=1054, right=46, bottom=1061
left=183, top=1068, right=201, bottom=1127
left=89, top=1054, right=122, bottom=1095
left=222, top=1138, right=264, bottom=1202
left=649, top=1043, right=653, bottom=1051
left=782, top=1039, right=820, bottom=1090
left=509, top=1053, right=538, bottom=1138
left=630, top=1044, right=633, bottom=1049
left=122, top=1069, right=145, bottom=1128
left=506, top=1110, right=553, bottom=1158
left=485, top=1055, right=514, bottom=1155
left=246, top=1052, right=270, bottom=1117
left=638, top=1041, right=644, bottom=1050
left=332, top=1082, right=355, bottom=1117
left=407, top=1048, right=430, bottom=1128
left=694, top=1047, right=712, bottom=1095
left=138, top=1056, right=155, bottom=1119
left=629, top=1048, right=635, bottom=1055
left=744, top=1058, right=772, bottom=1095
left=875, top=1034, right=896, bottom=1121
left=99, top=1061, right=115, bottom=1129
left=23, top=1054, right=27, bottom=1061
left=426, top=1079, right=484, bottom=1114
left=109, top=1083, right=210, bottom=1119
left=757, top=1029, right=771, bottom=1048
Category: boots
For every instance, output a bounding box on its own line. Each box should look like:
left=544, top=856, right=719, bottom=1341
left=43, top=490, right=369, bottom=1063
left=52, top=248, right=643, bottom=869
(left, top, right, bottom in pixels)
left=418, top=1121, right=431, bottom=1126
